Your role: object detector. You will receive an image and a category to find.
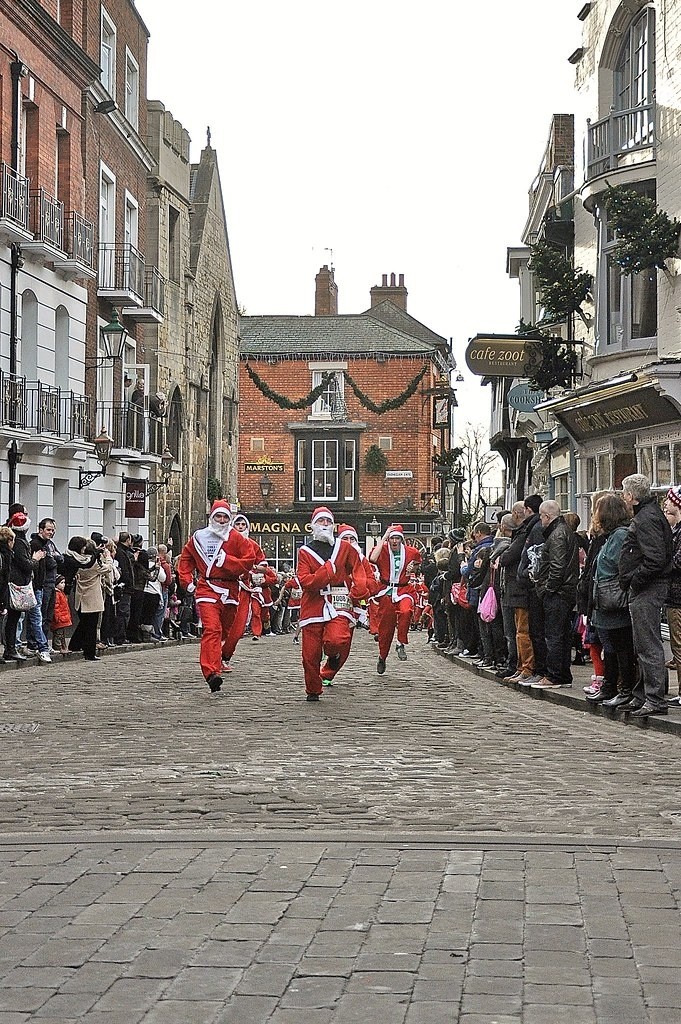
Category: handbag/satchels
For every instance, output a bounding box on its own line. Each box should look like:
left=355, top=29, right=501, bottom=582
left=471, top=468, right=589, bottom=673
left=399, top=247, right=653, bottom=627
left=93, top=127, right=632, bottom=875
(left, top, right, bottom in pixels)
left=465, top=586, right=482, bottom=609
left=8, top=581, right=39, bottom=612
left=593, top=579, right=628, bottom=614
left=477, top=567, right=498, bottom=623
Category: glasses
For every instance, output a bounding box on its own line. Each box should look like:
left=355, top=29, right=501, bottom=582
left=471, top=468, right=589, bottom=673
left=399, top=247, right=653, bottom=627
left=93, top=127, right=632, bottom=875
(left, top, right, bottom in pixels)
left=23, top=512, right=29, bottom=516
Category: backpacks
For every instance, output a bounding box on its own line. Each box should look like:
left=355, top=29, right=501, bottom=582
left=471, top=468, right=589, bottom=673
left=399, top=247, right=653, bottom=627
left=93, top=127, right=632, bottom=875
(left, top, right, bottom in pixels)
left=449, top=575, right=472, bottom=611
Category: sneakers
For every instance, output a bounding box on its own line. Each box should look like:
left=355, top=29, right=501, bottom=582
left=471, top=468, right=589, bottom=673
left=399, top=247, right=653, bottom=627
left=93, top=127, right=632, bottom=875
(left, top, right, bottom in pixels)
left=306, top=693, right=320, bottom=702
left=322, top=677, right=333, bottom=687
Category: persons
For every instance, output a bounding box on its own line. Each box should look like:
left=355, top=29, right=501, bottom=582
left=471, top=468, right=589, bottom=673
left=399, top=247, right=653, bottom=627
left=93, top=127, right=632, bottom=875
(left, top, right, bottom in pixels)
left=179, top=499, right=256, bottom=694
left=320, top=524, right=380, bottom=686
left=0, top=503, right=201, bottom=667
left=424, top=473, right=681, bottom=717
left=220, top=514, right=302, bottom=675
left=131, top=379, right=169, bottom=418
left=368, top=526, right=433, bottom=674
left=297, top=507, right=370, bottom=702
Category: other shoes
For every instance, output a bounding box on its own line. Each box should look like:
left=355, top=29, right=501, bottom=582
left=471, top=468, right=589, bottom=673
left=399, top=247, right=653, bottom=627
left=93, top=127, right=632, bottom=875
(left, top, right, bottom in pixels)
left=0, top=630, right=196, bottom=664
left=395, top=643, right=408, bottom=661
left=221, top=661, right=234, bottom=673
left=428, top=633, right=681, bottom=719
left=376, top=655, right=386, bottom=674
left=208, top=676, right=224, bottom=693
left=244, top=625, right=302, bottom=645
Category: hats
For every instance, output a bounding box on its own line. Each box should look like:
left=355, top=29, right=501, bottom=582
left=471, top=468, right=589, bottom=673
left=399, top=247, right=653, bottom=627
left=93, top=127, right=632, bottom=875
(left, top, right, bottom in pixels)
left=55, top=574, right=66, bottom=587
left=210, top=499, right=232, bottom=521
left=448, top=528, right=467, bottom=544
left=338, top=523, right=358, bottom=543
left=258, top=558, right=269, bottom=568
left=387, top=524, right=404, bottom=541
left=133, top=533, right=142, bottom=543
left=231, top=514, right=249, bottom=529
left=7, top=512, right=31, bottom=531
left=667, top=485, right=681, bottom=510
left=311, top=507, right=334, bottom=526
